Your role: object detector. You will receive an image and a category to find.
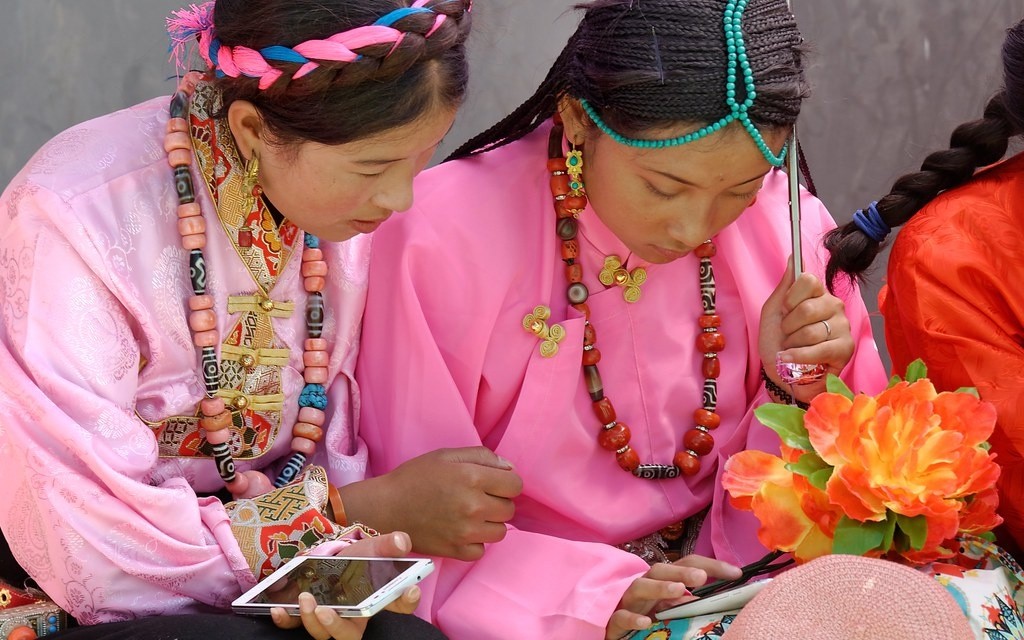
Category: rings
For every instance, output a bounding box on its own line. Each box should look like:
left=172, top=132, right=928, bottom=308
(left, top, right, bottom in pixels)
left=822, top=321, right=831, bottom=335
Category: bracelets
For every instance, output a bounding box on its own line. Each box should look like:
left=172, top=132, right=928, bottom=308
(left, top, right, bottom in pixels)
left=761, top=368, right=811, bottom=410
left=326, top=481, right=348, bottom=528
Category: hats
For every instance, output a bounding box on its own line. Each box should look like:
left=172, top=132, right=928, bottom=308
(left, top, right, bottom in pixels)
left=726, top=553, right=978, bottom=640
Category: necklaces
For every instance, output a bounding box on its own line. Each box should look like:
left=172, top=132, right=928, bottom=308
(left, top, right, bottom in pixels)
left=548, top=122, right=724, bottom=479
left=164, top=71, right=330, bottom=499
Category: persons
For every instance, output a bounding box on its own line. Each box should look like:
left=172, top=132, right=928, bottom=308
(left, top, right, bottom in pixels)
left=0, top=0, right=521, bottom=640
left=356, top=0, right=890, bottom=640
left=825, top=15, right=1024, bottom=559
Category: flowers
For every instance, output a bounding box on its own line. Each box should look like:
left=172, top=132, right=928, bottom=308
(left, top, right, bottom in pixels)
left=717, top=358, right=1005, bottom=566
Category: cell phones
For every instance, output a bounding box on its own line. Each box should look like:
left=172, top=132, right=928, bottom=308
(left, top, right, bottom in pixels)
left=655, top=579, right=773, bottom=620
left=231, top=555, right=435, bottom=618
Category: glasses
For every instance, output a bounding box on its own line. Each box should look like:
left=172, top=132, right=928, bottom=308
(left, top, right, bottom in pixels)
left=692, top=550, right=795, bottom=595
